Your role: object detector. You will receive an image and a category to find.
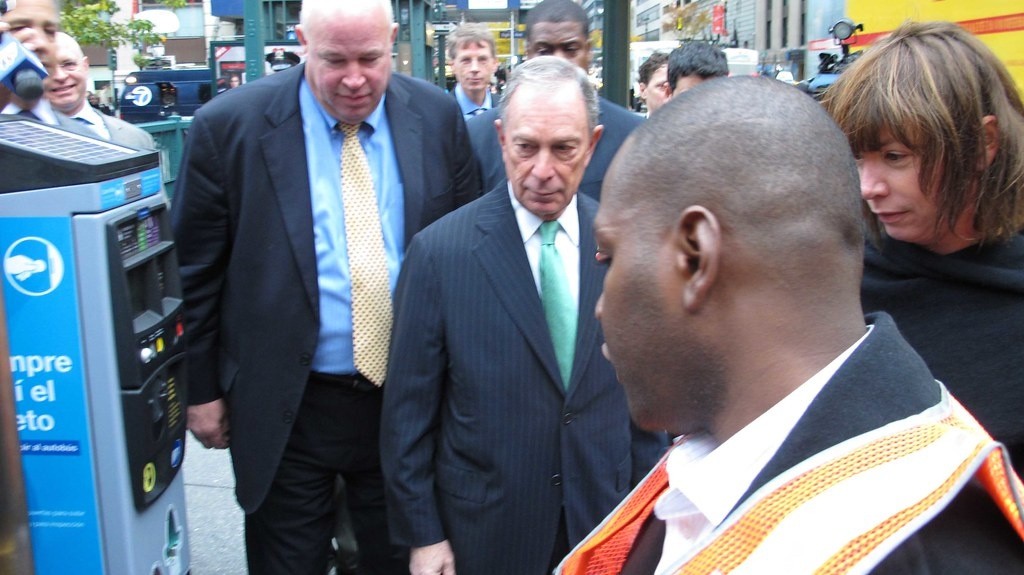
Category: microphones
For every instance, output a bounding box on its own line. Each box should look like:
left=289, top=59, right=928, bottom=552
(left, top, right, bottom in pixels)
left=0, top=29, right=49, bottom=101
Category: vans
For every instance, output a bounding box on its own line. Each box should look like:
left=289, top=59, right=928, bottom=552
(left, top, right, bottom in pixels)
left=120, top=68, right=218, bottom=125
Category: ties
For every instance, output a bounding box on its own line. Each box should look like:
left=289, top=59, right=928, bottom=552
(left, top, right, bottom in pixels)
left=475, top=110, right=484, bottom=115
left=337, top=121, right=396, bottom=388
left=537, top=221, right=578, bottom=392
left=19, top=110, right=35, bottom=119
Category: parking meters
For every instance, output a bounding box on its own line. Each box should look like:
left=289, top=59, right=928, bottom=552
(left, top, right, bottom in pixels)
left=0, top=110, right=194, bottom=575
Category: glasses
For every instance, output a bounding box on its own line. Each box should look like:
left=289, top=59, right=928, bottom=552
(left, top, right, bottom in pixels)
left=56, top=57, right=83, bottom=70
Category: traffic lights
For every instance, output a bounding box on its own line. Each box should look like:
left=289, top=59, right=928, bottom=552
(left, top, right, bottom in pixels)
left=108, top=47, right=117, bottom=72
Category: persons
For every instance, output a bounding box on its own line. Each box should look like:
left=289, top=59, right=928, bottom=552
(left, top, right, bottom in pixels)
left=819, top=22, right=1023, bottom=481
left=0, top=0, right=156, bottom=153
left=170, top=1, right=485, bottom=574
left=668, top=42, right=729, bottom=119
left=638, top=51, right=669, bottom=116
left=447, top=22, right=501, bottom=122
left=380, top=55, right=672, bottom=575
left=465, top=1, right=649, bottom=204
left=550, top=76, right=1023, bottom=574
